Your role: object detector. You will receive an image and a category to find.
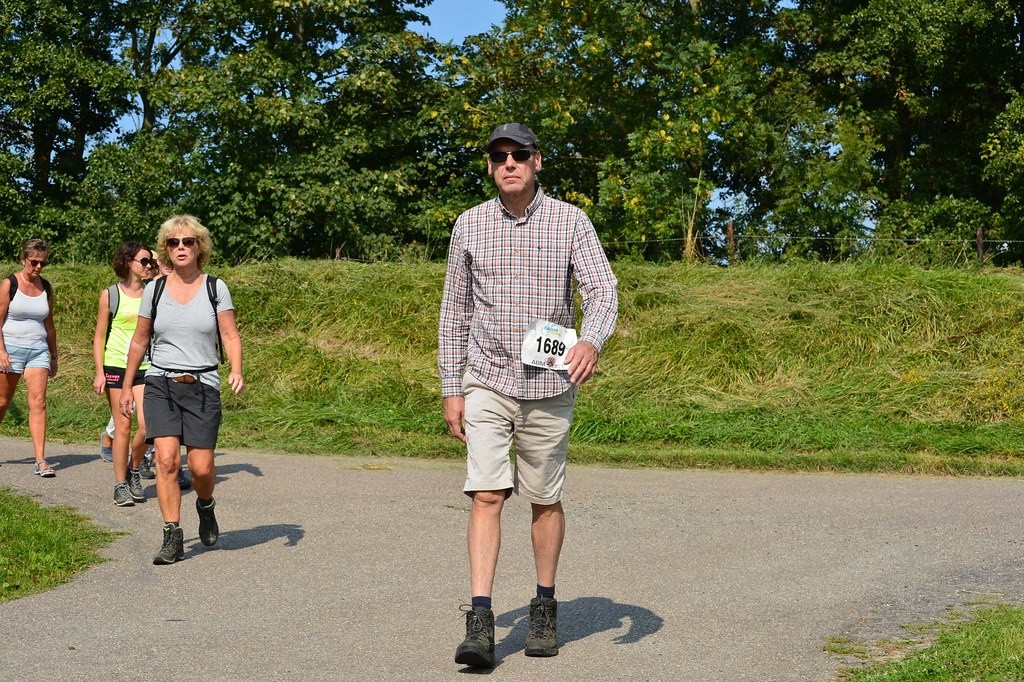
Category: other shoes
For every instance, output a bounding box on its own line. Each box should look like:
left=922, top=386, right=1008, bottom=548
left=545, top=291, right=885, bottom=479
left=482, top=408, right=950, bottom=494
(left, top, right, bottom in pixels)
left=100, top=432, right=113, bottom=462
left=145, top=459, right=155, bottom=467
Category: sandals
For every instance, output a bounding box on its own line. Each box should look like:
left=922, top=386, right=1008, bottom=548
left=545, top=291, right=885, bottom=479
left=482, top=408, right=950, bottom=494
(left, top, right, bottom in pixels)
left=34, top=460, right=55, bottom=477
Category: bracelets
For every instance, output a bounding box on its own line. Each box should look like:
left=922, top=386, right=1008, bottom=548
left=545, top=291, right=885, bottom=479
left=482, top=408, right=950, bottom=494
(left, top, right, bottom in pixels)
left=51, top=353, right=57, bottom=359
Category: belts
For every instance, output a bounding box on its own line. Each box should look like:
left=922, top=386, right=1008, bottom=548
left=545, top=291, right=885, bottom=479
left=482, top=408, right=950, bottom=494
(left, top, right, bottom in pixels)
left=171, top=375, right=196, bottom=384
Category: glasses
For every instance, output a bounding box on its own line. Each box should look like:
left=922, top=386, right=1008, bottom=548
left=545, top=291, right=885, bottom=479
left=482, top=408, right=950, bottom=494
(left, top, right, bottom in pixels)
left=132, top=257, right=154, bottom=266
left=24, top=255, right=46, bottom=267
left=166, top=236, right=200, bottom=248
left=489, top=149, right=535, bottom=163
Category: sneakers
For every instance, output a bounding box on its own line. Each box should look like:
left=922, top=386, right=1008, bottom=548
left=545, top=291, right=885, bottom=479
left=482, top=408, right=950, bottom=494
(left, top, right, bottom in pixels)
left=113, top=483, right=134, bottom=506
left=455, top=603, right=497, bottom=669
left=525, top=594, right=558, bottom=657
left=195, top=495, right=219, bottom=546
left=126, top=464, right=146, bottom=500
left=178, top=467, right=191, bottom=490
left=152, top=523, right=185, bottom=565
left=139, top=456, right=156, bottom=479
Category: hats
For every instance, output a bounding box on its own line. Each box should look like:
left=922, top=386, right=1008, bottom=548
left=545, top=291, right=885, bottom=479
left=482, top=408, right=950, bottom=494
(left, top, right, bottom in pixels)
left=487, top=123, right=538, bottom=151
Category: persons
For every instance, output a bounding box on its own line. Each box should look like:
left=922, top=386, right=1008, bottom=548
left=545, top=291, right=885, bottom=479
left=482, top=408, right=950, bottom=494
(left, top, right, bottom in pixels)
left=0, top=239, right=58, bottom=477
left=92, top=240, right=191, bottom=507
left=118, top=214, right=245, bottom=565
left=438, top=123, right=620, bottom=667
